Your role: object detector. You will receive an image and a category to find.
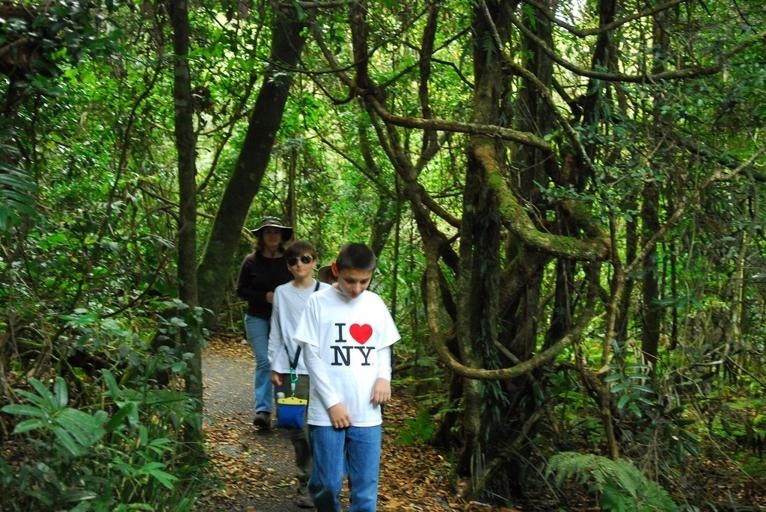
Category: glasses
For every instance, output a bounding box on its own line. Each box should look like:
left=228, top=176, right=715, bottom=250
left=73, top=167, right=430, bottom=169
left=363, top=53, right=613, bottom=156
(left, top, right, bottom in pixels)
left=287, top=255, right=312, bottom=266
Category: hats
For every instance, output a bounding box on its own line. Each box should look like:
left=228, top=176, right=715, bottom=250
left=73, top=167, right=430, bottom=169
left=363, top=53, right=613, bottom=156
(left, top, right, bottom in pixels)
left=249, top=216, right=293, bottom=241
left=318, top=260, right=335, bottom=282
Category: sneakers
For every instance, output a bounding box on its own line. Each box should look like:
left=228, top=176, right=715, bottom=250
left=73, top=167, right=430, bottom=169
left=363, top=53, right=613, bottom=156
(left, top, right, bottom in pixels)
left=252, top=411, right=270, bottom=428
left=295, top=488, right=313, bottom=509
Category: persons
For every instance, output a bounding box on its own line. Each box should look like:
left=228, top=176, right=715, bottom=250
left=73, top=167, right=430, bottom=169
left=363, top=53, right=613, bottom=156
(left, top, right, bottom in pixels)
left=235, top=213, right=294, bottom=432
left=267, top=238, right=333, bottom=506
left=292, top=241, right=402, bottom=512
left=316, top=259, right=338, bottom=286
